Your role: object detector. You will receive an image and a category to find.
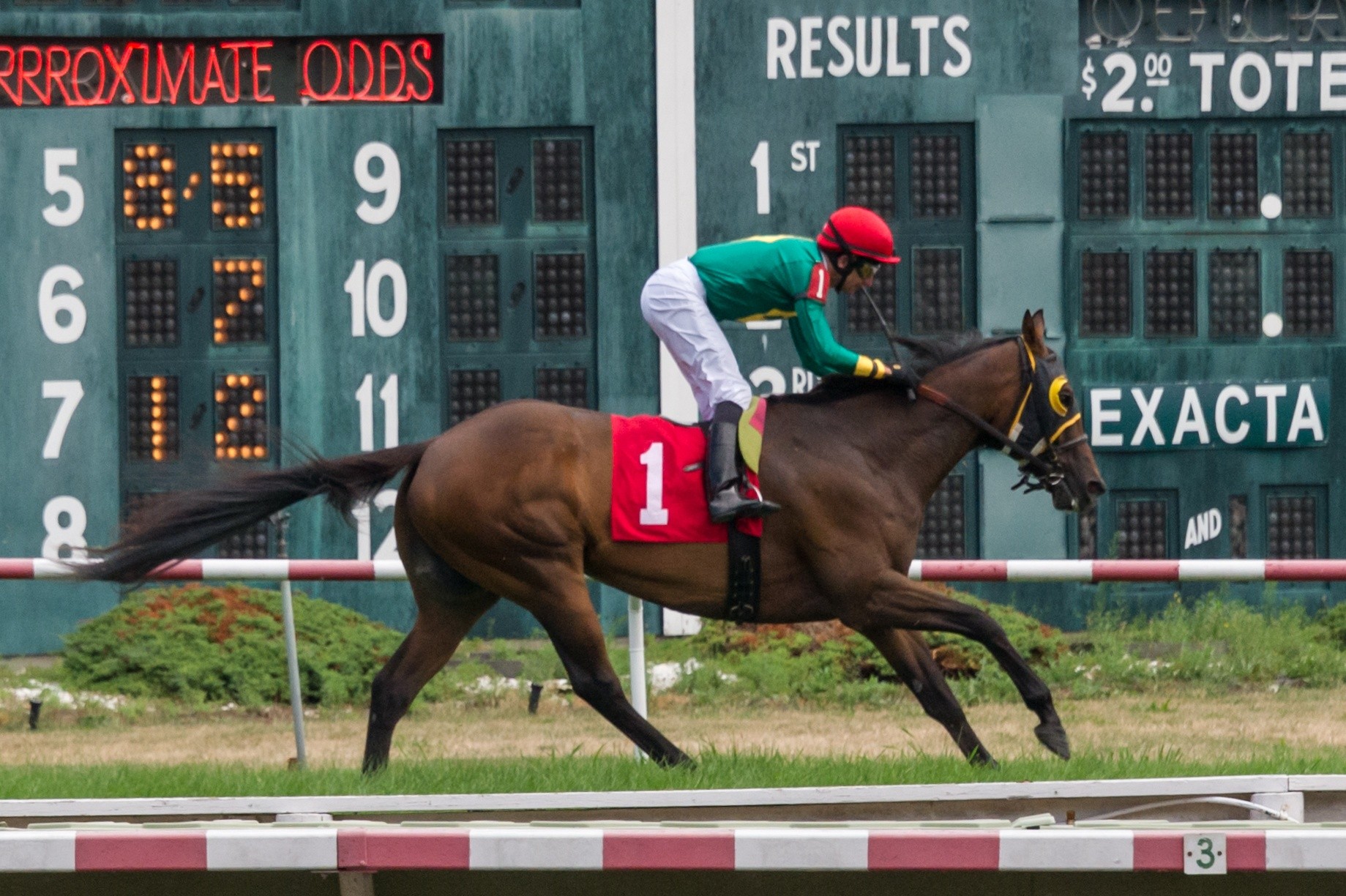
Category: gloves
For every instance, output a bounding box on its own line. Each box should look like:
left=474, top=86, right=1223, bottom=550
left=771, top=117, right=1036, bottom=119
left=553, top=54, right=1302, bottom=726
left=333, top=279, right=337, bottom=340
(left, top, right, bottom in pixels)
left=883, top=360, right=920, bottom=395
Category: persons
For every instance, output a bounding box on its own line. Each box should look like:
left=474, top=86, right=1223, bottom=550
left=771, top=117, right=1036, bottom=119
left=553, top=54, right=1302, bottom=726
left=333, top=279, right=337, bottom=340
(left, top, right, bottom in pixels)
left=641, top=206, right=901, bottom=522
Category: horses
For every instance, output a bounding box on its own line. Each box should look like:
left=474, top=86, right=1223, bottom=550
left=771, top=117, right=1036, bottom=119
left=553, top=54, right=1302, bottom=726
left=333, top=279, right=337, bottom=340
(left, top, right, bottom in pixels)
left=42, top=301, right=1099, bottom=770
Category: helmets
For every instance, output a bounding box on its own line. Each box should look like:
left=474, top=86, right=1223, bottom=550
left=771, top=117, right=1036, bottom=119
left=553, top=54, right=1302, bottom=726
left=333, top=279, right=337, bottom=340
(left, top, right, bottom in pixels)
left=816, top=207, right=901, bottom=264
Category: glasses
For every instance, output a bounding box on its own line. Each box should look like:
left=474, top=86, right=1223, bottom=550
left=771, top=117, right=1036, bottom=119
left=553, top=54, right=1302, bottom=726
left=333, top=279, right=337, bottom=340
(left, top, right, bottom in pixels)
left=858, top=261, right=881, bottom=280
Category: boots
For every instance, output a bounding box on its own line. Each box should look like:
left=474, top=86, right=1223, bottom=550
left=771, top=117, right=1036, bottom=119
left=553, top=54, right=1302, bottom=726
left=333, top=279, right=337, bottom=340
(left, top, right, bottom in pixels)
left=708, top=400, right=780, bottom=524
